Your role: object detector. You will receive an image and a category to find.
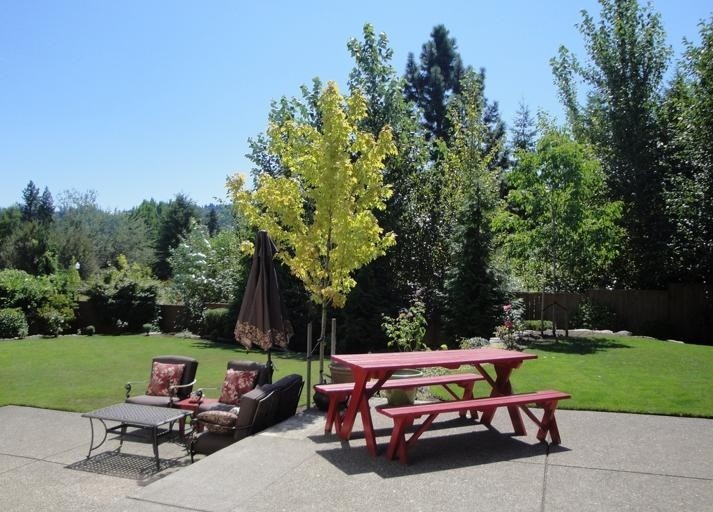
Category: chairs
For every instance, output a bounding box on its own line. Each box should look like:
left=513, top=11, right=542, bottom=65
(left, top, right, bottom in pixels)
left=123, top=354, right=303, bottom=463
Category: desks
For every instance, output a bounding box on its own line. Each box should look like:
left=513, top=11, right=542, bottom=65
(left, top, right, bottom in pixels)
left=329, top=346, right=538, bottom=458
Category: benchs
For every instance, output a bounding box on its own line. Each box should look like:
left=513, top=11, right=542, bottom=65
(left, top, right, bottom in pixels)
left=311, top=372, right=572, bottom=466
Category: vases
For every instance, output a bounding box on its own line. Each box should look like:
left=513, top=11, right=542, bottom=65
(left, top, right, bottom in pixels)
left=386, top=368, right=423, bottom=406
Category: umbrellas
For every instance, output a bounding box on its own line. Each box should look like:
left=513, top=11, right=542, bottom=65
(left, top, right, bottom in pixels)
left=234, top=230, right=292, bottom=384
